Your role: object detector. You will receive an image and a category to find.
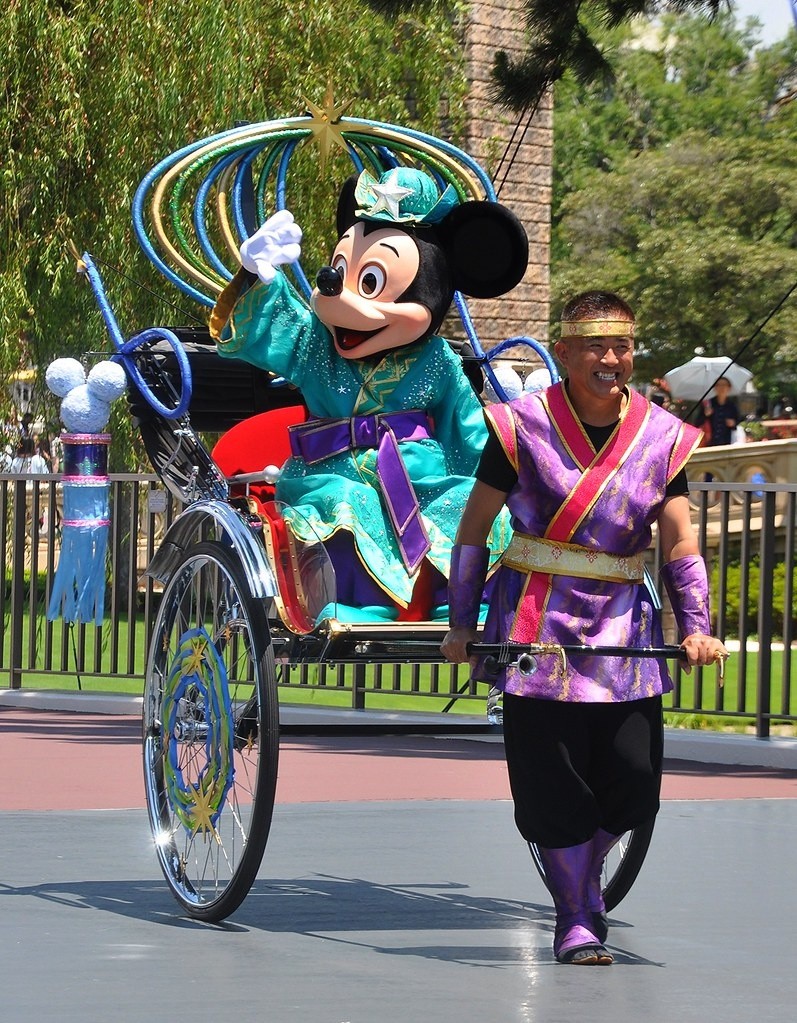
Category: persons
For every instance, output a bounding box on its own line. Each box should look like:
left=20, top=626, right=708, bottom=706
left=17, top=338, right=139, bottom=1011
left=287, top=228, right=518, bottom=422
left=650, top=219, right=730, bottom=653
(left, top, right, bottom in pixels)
left=694, top=377, right=738, bottom=446
left=439, top=293, right=727, bottom=962
left=0, top=430, right=67, bottom=545
left=653, top=392, right=670, bottom=410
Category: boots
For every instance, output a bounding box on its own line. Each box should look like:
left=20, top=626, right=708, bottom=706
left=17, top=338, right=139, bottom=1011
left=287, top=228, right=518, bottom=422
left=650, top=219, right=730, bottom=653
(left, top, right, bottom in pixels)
left=538, top=839, right=615, bottom=962
left=586, top=828, right=625, bottom=943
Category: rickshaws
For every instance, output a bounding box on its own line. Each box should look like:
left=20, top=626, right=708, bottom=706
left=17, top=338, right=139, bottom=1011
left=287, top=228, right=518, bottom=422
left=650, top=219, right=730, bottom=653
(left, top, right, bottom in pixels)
left=116, top=324, right=731, bottom=926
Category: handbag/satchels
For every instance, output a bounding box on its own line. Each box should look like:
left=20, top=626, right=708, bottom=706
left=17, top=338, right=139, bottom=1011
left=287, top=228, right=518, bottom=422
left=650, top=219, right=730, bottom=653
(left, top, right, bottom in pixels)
left=731, top=425, right=746, bottom=446
left=697, top=420, right=712, bottom=448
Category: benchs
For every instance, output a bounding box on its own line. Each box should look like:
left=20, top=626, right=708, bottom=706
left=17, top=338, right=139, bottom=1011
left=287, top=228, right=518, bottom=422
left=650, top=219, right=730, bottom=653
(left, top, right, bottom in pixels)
left=129, top=325, right=487, bottom=531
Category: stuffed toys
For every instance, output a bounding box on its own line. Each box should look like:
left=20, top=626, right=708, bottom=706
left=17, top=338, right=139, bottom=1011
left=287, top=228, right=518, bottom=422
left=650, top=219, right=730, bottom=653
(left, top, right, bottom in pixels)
left=208, top=168, right=529, bottom=622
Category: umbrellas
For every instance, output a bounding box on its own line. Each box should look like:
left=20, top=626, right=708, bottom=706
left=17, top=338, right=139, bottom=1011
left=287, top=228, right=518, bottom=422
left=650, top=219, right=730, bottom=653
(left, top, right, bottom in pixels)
left=662, top=356, right=754, bottom=402
